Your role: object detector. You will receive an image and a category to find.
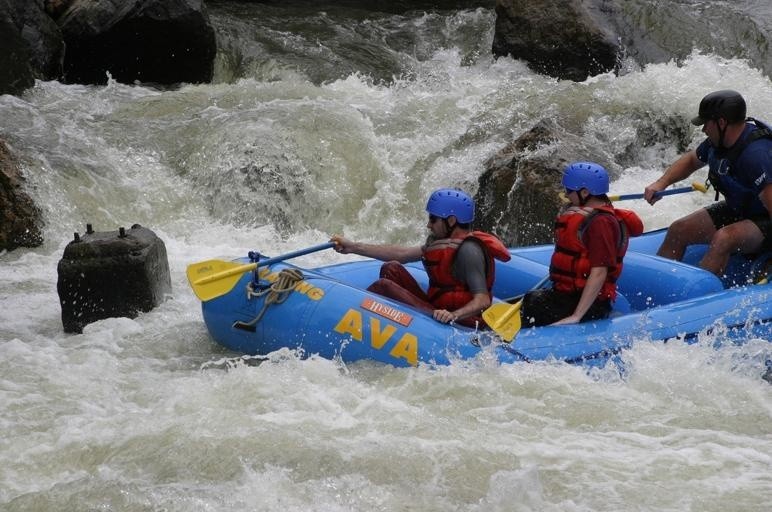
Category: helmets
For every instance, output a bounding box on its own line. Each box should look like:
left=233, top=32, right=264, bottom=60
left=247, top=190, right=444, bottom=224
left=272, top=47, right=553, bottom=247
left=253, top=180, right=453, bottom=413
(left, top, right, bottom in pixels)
left=425, top=189, right=476, bottom=224
left=561, top=162, right=609, bottom=196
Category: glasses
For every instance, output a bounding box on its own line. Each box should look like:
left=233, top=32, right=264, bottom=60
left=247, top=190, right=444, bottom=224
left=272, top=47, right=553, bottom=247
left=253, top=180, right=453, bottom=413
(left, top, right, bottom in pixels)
left=429, top=214, right=436, bottom=224
left=565, top=187, right=574, bottom=195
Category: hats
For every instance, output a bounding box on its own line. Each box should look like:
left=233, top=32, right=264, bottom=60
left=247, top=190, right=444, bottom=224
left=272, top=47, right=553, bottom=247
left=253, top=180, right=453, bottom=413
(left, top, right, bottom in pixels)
left=691, top=89, right=746, bottom=126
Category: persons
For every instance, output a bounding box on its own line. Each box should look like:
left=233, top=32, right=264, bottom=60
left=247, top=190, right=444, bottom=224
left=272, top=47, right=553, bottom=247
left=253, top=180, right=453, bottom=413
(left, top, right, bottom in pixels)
left=328, top=189, right=495, bottom=325
left=644, top=90, right=772, bottom=277
left=523, top=162, right=629, bottom=327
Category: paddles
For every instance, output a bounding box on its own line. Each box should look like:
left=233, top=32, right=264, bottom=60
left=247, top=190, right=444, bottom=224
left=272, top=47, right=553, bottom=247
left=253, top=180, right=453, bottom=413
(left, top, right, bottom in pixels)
left=559, top=182, right=708, bottom=205
left=186, top=240, right=339, bottom=301
left=482, top=272, right=550, bottom=342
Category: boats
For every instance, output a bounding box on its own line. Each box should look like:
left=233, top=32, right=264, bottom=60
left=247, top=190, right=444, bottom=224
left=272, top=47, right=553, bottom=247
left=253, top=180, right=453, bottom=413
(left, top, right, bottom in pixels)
left=202, top=202, right=771, bottom=366
